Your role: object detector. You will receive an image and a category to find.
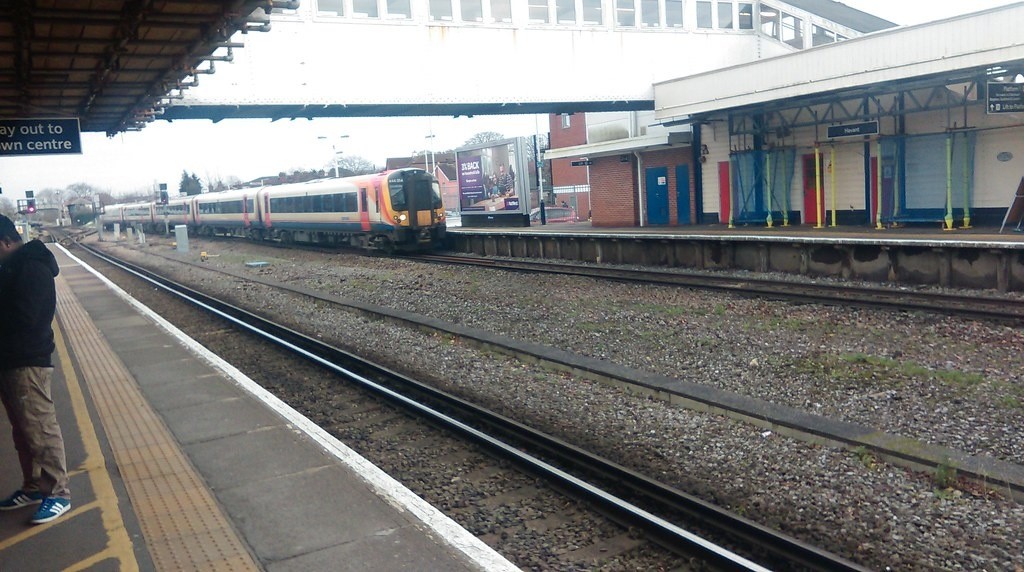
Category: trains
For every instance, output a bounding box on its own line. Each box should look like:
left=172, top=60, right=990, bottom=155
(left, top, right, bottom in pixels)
left=97, top=167, right=448, bottom=255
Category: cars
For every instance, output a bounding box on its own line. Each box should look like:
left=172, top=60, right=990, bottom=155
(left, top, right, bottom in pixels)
left=529, top=206, right=576, bottom=226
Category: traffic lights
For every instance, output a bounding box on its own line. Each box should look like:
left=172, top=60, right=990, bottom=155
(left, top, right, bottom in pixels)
left=27, top=199, right=37, bottom=213
left=161, top=191, right=169, bottom=204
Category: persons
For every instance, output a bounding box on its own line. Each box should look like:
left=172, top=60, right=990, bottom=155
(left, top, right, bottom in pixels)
left=482, top=163, right=515, bottom=198
left=0, top=214, right=71, bottom=524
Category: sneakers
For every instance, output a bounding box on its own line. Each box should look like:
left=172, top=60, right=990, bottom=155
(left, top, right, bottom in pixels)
left=0, top=490, right=42, bottom=511
left=31, top=497, right=71, bottom=524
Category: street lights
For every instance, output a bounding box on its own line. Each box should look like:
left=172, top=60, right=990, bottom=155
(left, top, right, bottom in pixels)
left=423, top=134, right=436, bottom=172
left=318, top=134, right=351, bottom=178
